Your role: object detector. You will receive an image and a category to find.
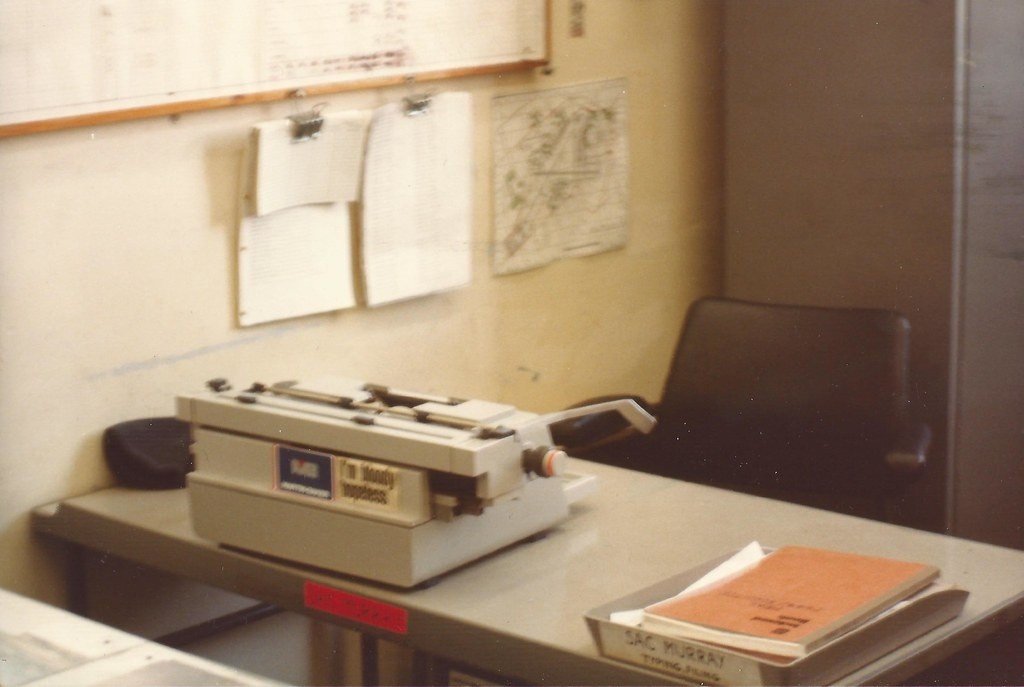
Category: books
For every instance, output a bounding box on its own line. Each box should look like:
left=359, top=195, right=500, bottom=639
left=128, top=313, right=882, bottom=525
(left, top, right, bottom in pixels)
left=639, top=546, right=942, bottom=658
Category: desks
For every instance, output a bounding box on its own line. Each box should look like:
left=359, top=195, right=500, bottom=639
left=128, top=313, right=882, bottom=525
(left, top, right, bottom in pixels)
left=33, top=448, right=1023, bottom=687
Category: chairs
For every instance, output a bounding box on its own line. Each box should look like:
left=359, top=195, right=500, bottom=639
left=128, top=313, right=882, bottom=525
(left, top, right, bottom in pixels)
left=540, top=297, right=935, bottom=526
